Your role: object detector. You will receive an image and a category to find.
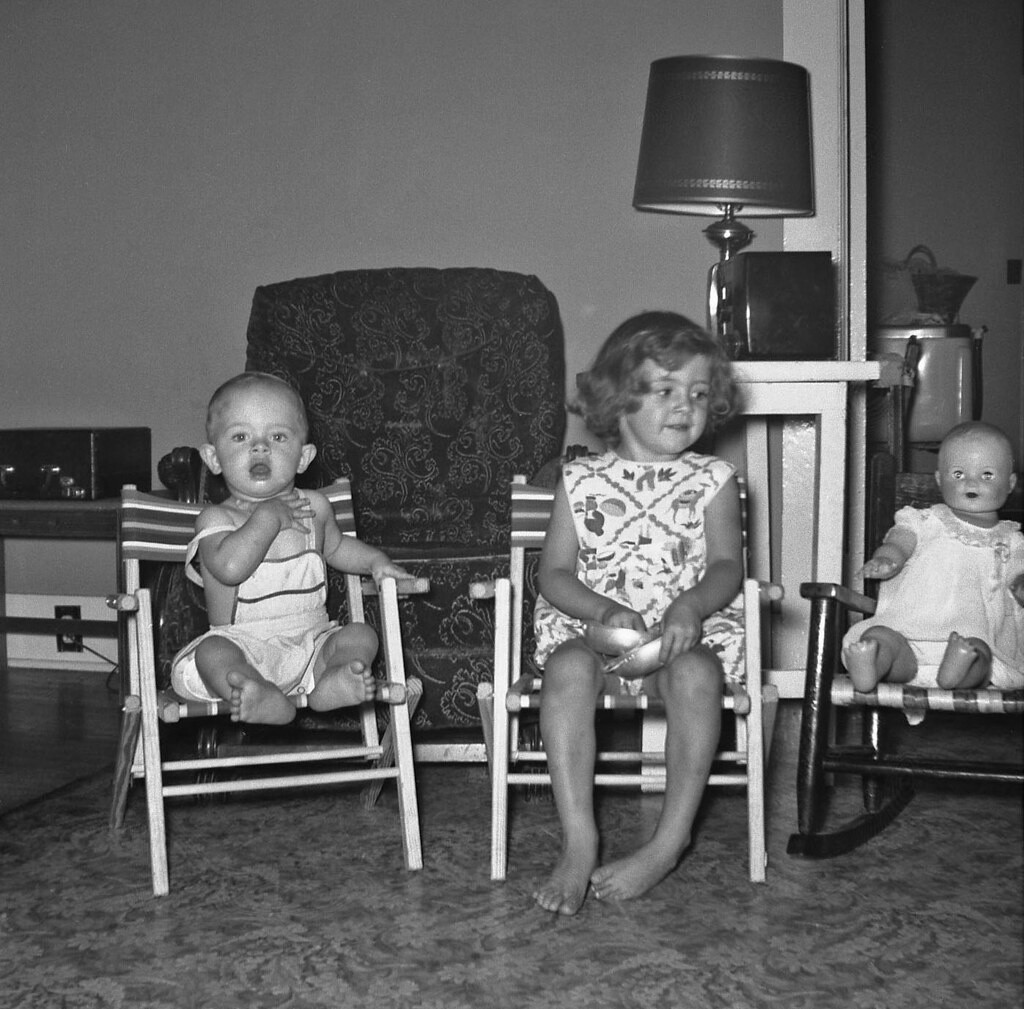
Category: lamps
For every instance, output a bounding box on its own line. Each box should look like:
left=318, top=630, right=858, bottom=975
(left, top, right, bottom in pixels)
left=632, top=55, right=815, bottom=363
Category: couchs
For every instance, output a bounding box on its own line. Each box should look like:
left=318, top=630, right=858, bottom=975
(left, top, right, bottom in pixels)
left=157, top=270, right=578, bottom=806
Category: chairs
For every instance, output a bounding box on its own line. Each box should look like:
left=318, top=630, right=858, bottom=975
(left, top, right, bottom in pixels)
left=787, top=581, right=1024, bottom=859
left=468, top=476, right=786, bottom=881
left=108, top=476, right=429, bottom=896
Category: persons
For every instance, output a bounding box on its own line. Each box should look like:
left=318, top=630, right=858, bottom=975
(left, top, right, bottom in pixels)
left=841, top=420, right=1024, bottom=693
left=170, top=372, right=414, bottom=727
left=531, top=311, right=747, bottom=916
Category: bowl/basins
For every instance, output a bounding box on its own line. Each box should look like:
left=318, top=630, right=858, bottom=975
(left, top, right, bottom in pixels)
left=582, top=624, right=652, bottom=656
left=604, top=631, right=667, bottom=679
